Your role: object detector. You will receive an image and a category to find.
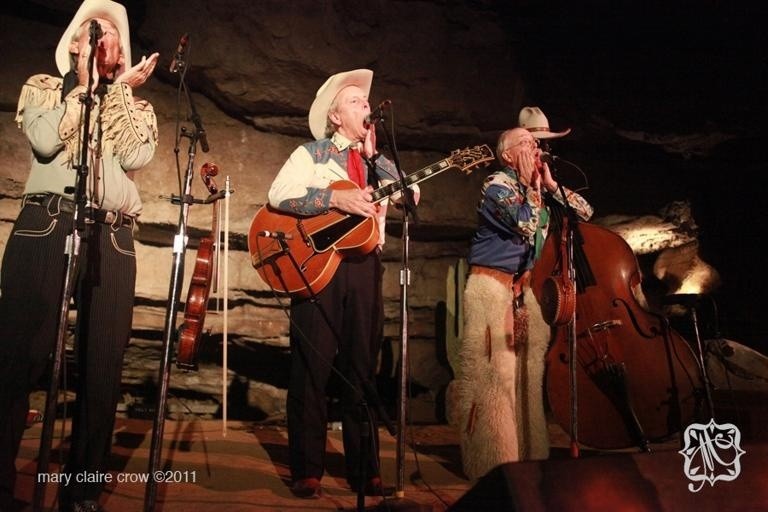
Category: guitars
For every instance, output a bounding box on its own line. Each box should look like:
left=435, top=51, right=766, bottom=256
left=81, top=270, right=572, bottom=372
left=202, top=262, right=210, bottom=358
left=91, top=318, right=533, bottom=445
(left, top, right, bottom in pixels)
left=248, top=145, right=496, bottom=300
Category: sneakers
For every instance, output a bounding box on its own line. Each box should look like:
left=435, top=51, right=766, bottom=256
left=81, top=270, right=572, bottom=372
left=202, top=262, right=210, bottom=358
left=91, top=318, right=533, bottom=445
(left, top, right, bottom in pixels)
left=350, top=476, right=393, bottom=497
left=292, top=477, right=323, bottom=500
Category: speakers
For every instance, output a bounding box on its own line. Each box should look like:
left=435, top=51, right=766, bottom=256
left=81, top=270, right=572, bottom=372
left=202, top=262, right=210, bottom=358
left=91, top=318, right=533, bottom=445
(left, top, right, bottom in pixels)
left=448, top=441, right=768, bottom=512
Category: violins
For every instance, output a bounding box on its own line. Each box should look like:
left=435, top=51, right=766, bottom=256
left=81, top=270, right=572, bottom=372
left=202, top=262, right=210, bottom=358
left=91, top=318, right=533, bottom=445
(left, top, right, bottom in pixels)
left=176, top=162, right=220, bottom=366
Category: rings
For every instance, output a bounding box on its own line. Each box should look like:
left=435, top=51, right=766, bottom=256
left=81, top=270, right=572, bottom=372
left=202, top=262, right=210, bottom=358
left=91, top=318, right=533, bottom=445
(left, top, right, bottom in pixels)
left=81, top=54, right=89, bottom=59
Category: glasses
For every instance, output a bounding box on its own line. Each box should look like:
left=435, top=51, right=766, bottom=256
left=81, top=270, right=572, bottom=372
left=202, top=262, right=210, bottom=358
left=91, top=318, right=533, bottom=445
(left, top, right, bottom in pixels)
left=506, top=138, right=541, bottom=150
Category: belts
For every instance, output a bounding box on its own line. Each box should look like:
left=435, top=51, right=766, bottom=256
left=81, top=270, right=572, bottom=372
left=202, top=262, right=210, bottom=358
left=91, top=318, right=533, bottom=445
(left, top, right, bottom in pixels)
left=21, top=192, right=135, bottom=230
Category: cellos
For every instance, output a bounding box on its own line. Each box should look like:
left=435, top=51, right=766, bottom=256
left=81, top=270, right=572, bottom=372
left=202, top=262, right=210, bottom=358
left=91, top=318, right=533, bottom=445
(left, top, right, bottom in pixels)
left=531, top=146, right=706, bottom=448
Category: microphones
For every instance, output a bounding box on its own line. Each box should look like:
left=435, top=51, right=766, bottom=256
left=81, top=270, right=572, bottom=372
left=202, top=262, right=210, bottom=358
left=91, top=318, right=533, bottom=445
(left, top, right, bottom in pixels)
left=540, top=151, right=553, bottom=175
left=257, top=230, right=296, bottom=243
left=363, top=99, right=393, bottom=126
left=660, top=293, right=703, bottom=305
left=552, top=154, right=561, bottom=162
left=87, top=19, right=103, bottom=40
left=169, top=32, right=191, bottom=74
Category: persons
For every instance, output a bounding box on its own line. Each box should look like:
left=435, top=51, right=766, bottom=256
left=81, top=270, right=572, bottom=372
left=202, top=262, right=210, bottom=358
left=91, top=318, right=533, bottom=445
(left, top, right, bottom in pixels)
left=443, top=124, right=594, bottom=484
left=0, top=0, right=162, bottom=512
left=267, top=67, right=422, bottom=497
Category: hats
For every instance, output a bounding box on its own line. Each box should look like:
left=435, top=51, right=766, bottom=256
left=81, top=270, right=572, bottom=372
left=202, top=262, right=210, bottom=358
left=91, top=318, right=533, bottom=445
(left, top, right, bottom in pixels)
left=517, top=105, right=573, bottom=141
left=55, top=0, right=134, bottom=81
left=307, top=68, right=376, bottom=143
